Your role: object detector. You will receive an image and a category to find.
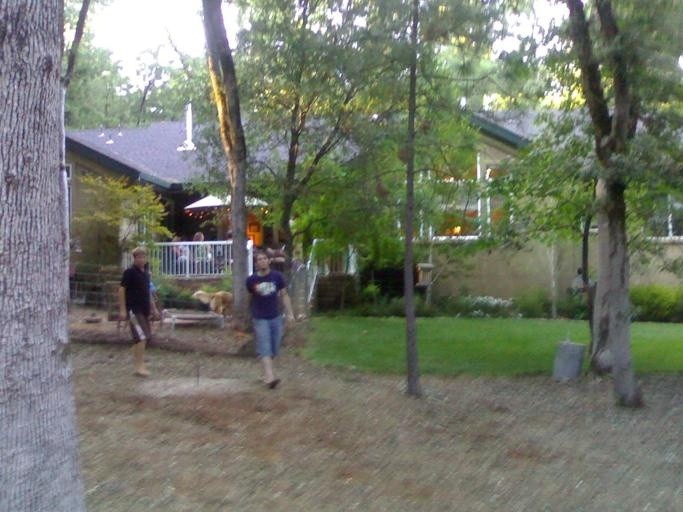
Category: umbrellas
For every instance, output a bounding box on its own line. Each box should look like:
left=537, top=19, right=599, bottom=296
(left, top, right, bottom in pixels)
left=183, top=193, right=269, bottom=212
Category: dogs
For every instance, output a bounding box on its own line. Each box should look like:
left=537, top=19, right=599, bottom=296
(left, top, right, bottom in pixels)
left=209, top=291, right=234, bottom=315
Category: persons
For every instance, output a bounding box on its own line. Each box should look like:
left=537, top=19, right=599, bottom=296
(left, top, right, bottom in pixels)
left=192, top=232, right=210, bottom=265
left=118, top=247, right=160, bottom=377
left=246, top=254, right=296, bottom=388
